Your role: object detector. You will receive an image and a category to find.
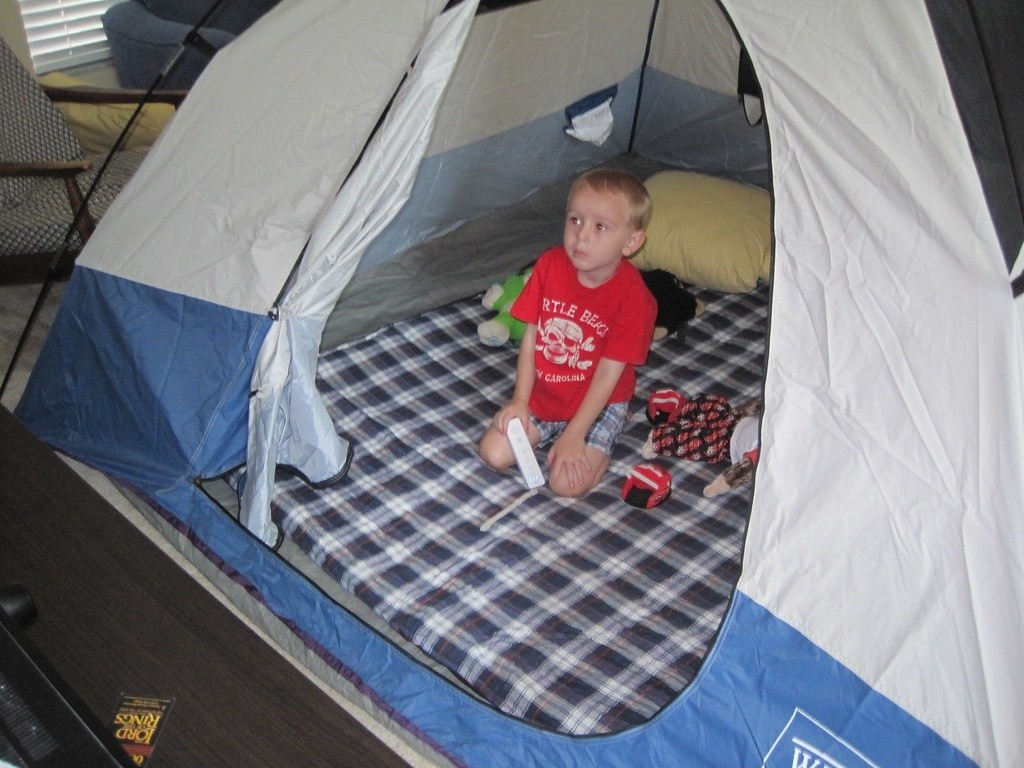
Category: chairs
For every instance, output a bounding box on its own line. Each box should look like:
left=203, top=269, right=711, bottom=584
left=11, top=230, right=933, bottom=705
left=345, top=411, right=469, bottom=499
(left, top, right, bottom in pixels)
left=0, top=37, right=191, bottom=287
left=100, top=0, right=279, bottom=90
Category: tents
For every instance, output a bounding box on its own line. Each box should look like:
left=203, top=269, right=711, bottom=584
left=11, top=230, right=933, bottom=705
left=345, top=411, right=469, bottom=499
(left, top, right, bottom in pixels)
left=0, top=0, right=1024, bottom=768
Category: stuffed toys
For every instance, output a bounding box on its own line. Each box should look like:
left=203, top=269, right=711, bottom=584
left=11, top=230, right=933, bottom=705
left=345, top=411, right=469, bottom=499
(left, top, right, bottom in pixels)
left=641, top=388, right=760, bottom=497
left=638, top=269, right=704, bottom=342
left=478, top=267, right=533, bottom=347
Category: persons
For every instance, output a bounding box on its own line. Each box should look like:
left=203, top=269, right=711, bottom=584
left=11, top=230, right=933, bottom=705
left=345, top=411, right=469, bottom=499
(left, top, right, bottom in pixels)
left=480, top=169, right=657, bottom=497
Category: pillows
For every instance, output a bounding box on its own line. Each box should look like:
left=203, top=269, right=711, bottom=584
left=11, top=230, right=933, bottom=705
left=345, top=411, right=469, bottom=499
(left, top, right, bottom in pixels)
left=627, top=171, right=773, bottom=294
